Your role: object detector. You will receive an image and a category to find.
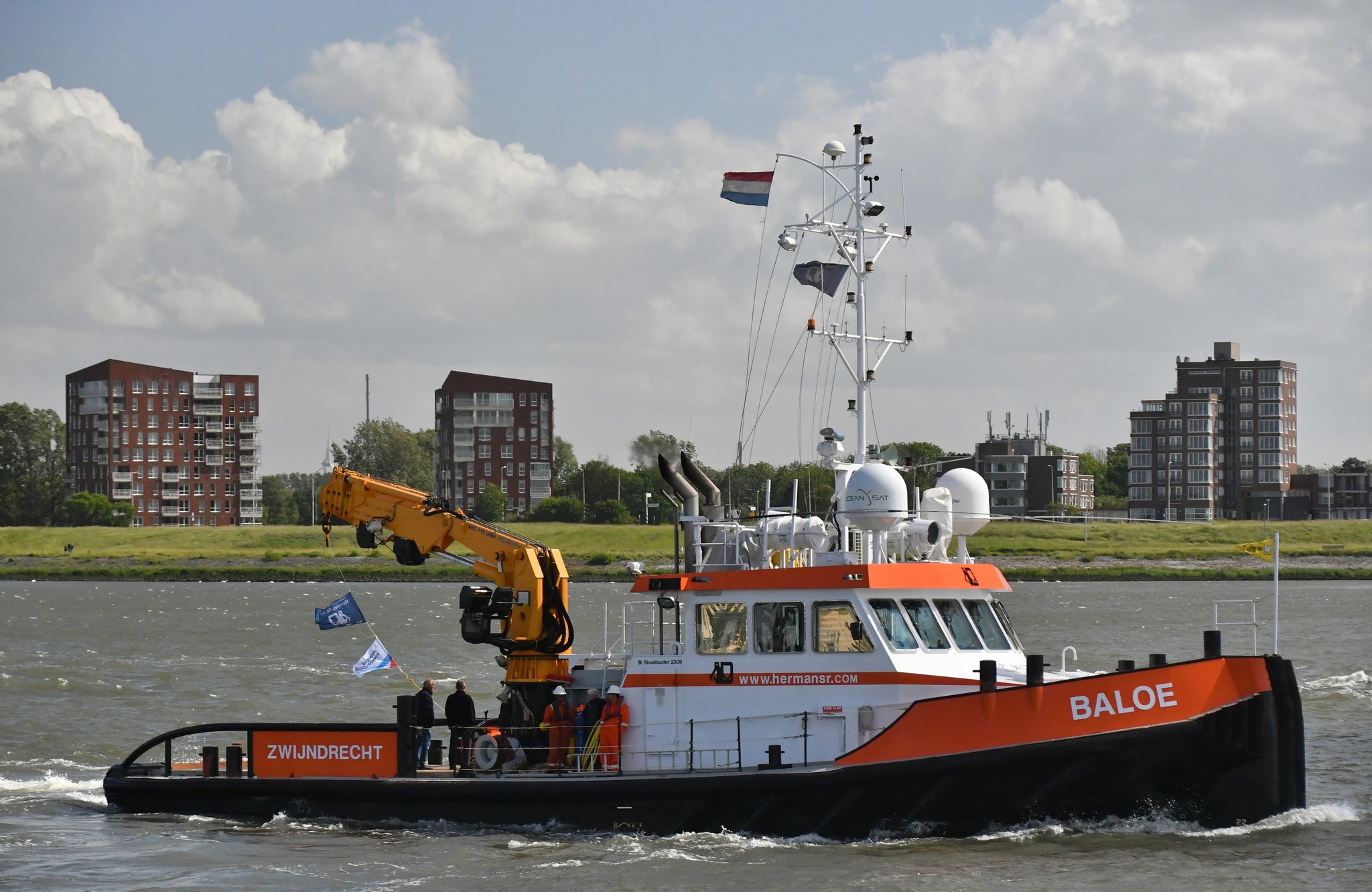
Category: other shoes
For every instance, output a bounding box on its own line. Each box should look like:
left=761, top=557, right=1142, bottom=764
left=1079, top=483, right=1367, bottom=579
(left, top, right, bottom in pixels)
left=593, top=765, right=599, bottom=771
left=416, top=764, right=433, bottom=770
left=543, top=773, right=569, bottom=778
left=596, top=775, right=614, bottom=777
left=449, top=766, right=457, bottom=770
left=460, top=766, right=474, bottom=771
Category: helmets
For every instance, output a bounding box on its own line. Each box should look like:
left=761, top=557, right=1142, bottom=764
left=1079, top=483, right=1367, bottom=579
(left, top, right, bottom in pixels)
left=606, top=685, right=621, bottom=694
left=553, top=686, right=567, bottom=695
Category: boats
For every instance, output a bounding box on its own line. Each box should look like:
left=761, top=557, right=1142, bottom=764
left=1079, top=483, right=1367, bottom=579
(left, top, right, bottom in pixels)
left=99, top=122, right=1309, bottom=832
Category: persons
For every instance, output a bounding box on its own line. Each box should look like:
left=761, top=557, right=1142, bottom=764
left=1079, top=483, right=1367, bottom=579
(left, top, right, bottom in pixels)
left=416, top=678, right=435, bottom=770
left=543, top=685, right=574, bottom=773
left=445, top=680, right=475, bottom=770
left=599, top=685, right=629, bottom=771
left=582, top=688, right=606, bottom=771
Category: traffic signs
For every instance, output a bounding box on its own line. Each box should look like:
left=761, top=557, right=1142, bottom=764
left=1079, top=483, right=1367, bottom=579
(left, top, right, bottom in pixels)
left=749, top=512, right=755, bottom=517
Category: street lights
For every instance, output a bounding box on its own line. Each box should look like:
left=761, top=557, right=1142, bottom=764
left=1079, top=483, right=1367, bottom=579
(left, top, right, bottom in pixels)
left=804, top=466, right=811, bottom=518
left=501, top=466, right=507, bottom=520
left=744, top=497, right=754, bottom=522
left=1168, top=461, right=1172, bottom=523
left=912, top=467, right=917, bottom=519
left=757, top=490, right=760, bottom=522
left=580, top=469, right=585, bottom=518
left=312, top=470, right=318, bottom=526
left=1266, top=499, right=1270, bottom=521
left=748, top=489, right=757, bottom=522
left=1263, top=503, right=1267, bottom=540
left=1085, top=500, right=1089, bottom=542
left=442, top=470, right=447, bottom=500
left=131, top=471, right=140, bottom=528
left=1324, top=463, right=1330, bottom=520
left=1048, top=464, right=1055, bottom=521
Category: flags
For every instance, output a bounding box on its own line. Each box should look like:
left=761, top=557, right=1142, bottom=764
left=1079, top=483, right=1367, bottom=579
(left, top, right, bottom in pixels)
left=353, top=639, right=398, bottom=679
left=314, top=592, right=366, bottom=630
